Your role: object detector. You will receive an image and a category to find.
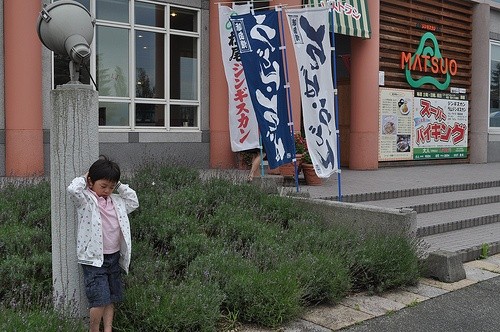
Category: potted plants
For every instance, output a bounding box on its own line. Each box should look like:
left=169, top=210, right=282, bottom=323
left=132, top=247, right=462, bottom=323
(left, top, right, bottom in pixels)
left=242, top=131, right=324, bottom=186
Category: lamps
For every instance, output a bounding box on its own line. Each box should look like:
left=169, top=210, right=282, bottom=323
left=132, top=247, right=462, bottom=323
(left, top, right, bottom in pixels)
left=36, top=0, right=95, bottom=90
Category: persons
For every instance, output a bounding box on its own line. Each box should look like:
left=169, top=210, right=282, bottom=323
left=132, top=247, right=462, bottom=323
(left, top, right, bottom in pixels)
left=247, top=148, right=266, bottom=182
left=67, top=154, right=140, bottom=332
